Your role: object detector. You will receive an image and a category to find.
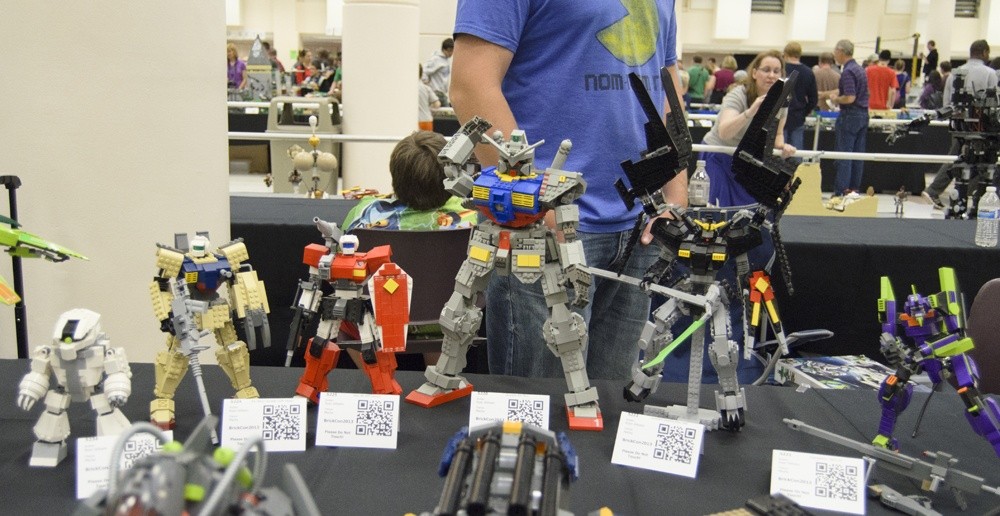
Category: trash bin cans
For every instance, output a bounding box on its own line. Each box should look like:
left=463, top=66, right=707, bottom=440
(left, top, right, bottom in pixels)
left=266, top=96, right=342, bottom=194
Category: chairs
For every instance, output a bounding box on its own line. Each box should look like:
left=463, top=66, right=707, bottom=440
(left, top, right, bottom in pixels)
left=335, top=216, right=491, bottom=372
left=960, top=275, right=1000, bottom=354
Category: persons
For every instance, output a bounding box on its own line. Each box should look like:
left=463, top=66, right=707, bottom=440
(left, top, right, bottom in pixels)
left=940, top=61, right=951, bottom=83
left=418, top=65, right=441, bottom=132
left=227, top=44, right=247, bottom=91
left=919, top=40, right=938, bottom=75
left=340, top=130, right=479, bottom=340
left=677, top=55, right=747, bottom=105
left=830, top=39, right=870, bottom=195
left=290, top=48, right=342, bottom=103
left=696, top=49, right=797, bottom=209
left=919, top=69, right=943, bottom=109
left=782, top=42, right=818, bottom=150
left=262, top=42, right=285, bottom=73
left=922, top=40, right=1000, bottom=209
left=450, top=0, right=688, bottom=381
left=863, top=49, right=912, bottom=110
left=425, top=38, right=454, bottom=107
left=812, top=51, right=841, bottom=111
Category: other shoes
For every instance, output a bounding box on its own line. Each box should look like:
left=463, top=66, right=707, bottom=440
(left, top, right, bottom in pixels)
left=921, top=188, right=944, bottom=209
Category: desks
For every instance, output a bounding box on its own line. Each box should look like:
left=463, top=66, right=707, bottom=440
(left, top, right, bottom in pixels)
left=0, top=356, right=1000, bottom=516
left=677, top=188, right=999, bottom=354
left=683, top=98, right=965, bottom=188
left=229, top=191, right=381, bottom=367
left=421, top=100, right=472, bottom=138
